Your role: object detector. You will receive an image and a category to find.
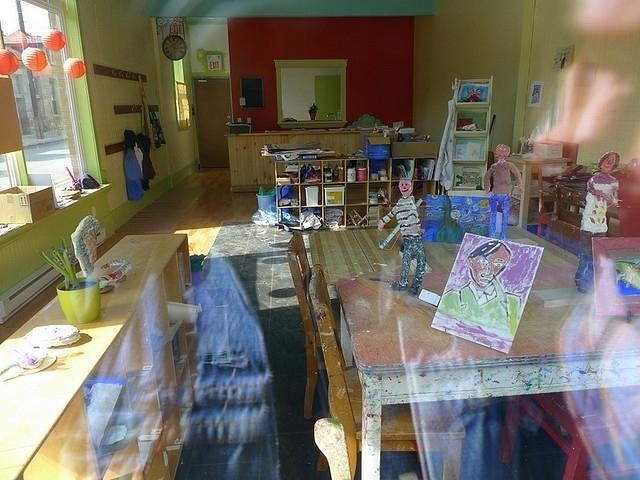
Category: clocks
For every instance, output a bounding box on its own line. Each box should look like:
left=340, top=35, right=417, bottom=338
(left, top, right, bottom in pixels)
left=162, top=35, right=187, bottom=61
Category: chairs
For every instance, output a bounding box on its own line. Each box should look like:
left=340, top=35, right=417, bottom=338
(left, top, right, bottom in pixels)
left=495, top=387, right=640, bottom=480
left=285, top=232, right=344, bottom=420
left=313, top=417, right=354, bottom=480
left=310, top=264, right=468, bottom=480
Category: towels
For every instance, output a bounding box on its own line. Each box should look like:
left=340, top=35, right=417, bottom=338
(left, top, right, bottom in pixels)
left=434, top=100, right=456, bottom=189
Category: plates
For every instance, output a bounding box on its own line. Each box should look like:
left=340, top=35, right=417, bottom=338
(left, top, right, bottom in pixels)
left=11, top=349, right=57, bottom=375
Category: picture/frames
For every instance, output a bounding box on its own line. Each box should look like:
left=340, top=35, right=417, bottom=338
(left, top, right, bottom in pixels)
left=527, top=80, right=544, bottom=107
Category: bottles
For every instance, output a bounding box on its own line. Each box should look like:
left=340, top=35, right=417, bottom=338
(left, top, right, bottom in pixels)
left=324, top=166, right=367, bottom=183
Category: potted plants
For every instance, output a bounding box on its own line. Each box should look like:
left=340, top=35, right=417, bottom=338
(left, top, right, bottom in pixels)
left=308, top=104, right=319, bottom=120
left=39, top=245, right=101, bottom=324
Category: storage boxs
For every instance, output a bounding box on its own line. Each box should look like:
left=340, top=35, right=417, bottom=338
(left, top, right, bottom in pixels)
left=362, top=131, right=391, bottom=159
left=0, top=185, right=55, bottom=225
left=324, top=185, right=345, bottom=206
left=304, top=186, right=320, bottom=208
left=391, top=139, right=439, bottom=158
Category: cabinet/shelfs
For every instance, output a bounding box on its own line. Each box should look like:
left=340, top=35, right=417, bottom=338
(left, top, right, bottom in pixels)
left=544, top=179, right=623, bottom=258
left=0, top=234, right=203, bottom=480
left=449, top=77, right=494, bottom=196
left=274, top=155, right=437, bottom=230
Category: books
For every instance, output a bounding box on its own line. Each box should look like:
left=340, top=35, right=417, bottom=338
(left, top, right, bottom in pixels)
left=366, top=204, right=386, bottom=228
left=416, top=160, right=436, bottom=181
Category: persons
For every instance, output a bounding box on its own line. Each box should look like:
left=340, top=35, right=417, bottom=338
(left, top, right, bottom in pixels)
left=440, top=241, right=521, bottom=341
left=469, top=88, right=484, bottom=103
left=559, top=51, right=569, bottom=67
left=460, top=87, right=472, bottom=103
left=571, top=150, right=639, bottom=297
left=484, top=142, right=525, bottom=240
left=377, top=177, right=428, bottom=299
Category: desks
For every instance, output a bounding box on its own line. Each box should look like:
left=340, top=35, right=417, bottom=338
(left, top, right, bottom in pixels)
left=509, top=156, right=575, bottom=229
left=309, top=222, right=639, bottom=478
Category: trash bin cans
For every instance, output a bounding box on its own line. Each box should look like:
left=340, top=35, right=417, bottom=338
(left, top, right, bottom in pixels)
left=256, top=193, right=276, bottom=212
